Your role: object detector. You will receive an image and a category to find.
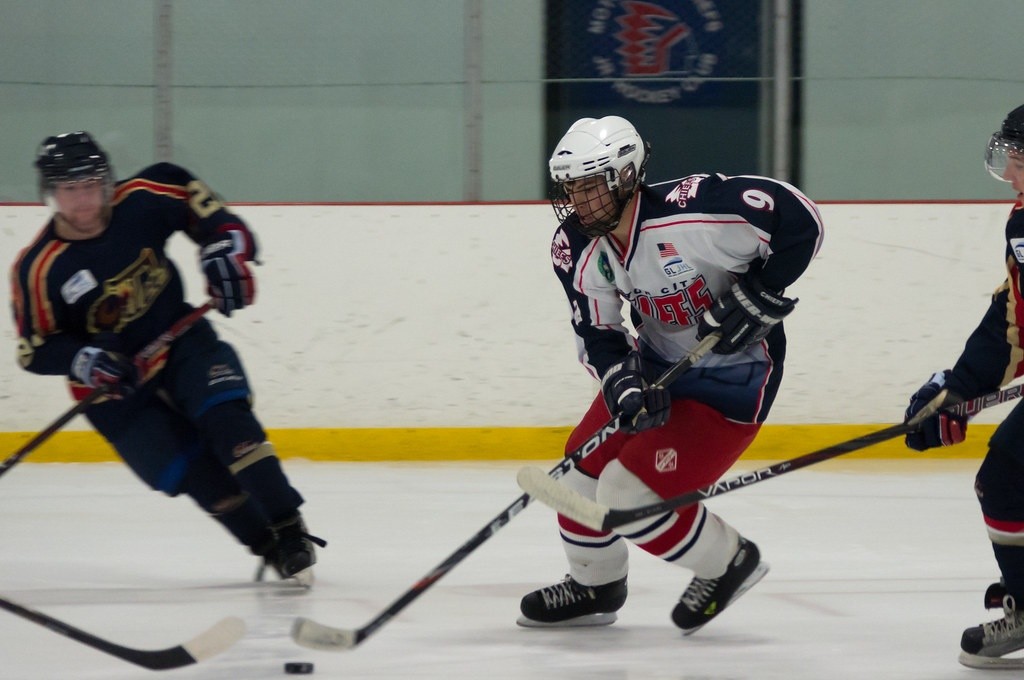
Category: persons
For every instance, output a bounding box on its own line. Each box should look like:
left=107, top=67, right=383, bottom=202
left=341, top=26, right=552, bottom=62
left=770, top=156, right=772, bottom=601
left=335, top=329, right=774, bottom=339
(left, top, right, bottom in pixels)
left=10, top=131, right=327, bottom=587
left=904, top=104, right=1023, bottom=670
left=517, top=117, right=825, bottom=637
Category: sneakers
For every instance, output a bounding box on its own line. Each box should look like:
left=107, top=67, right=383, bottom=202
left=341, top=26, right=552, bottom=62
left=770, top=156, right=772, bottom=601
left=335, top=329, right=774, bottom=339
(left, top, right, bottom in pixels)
left=960, top=584, right=1024, bottom=669
left=516, top=574, right=630, bottom=629
left=256, top=515, right=328, bottom=589
left=671, top=537, right=767, bottom=636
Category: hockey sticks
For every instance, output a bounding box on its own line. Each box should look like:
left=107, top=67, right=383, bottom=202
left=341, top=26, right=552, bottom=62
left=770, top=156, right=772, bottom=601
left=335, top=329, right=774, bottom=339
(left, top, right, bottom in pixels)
left=293, top=325, right=727, bottom=648
left=514, top=378, right=1023, bottom=534
left=0, top=295, right=220, bottom=477
left=0, top=596, right=244, bottom=672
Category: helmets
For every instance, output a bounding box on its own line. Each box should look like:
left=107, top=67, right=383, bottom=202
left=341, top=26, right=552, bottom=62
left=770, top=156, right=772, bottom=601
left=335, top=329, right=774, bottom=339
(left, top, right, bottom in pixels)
left=37, top=130, right=108, bottom=183
left=549, top=115, right=647, bottom=191
left=1001, top=105, right=1024, bottom=142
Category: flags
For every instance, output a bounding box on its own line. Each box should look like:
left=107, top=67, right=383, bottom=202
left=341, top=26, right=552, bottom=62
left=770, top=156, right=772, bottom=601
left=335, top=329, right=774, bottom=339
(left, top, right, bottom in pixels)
left=562, top=1, right=761, bottom=109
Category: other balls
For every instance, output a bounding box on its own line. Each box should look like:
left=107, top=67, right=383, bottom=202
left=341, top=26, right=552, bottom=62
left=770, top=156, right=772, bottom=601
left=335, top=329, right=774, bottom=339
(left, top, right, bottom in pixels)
left=285, top=662, right=315, bottom=674
